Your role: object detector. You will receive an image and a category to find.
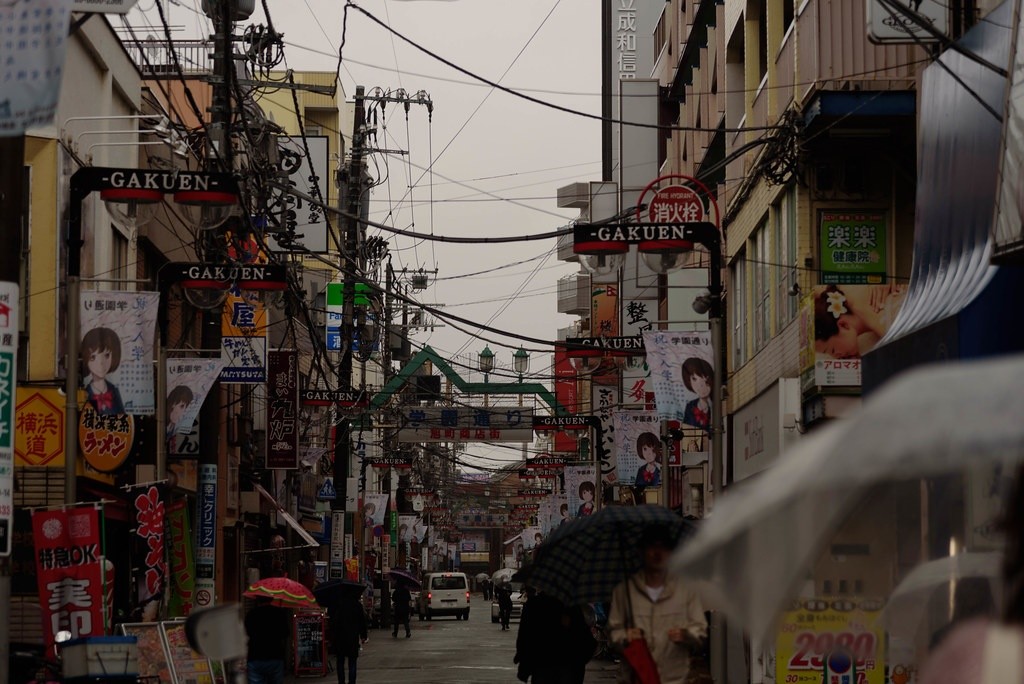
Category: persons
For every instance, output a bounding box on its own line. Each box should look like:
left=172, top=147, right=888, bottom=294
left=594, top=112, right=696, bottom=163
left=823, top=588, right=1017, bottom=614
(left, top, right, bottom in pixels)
left=915, top=456, right=1024, bottom=684
left=481, top=577, right=493, bottom=601
left=243, top=595, right=291, bottom=684
left=513, top=569, right=597, bottom=684
left=325, top=588, right=370, bottom=684
left=494, top=575, right=513, bottom=630
left=392, top=580, right=411, bottom=638
left=814, top=285, right=908, bottom=358
left=609, top=524, right=707, bottom=684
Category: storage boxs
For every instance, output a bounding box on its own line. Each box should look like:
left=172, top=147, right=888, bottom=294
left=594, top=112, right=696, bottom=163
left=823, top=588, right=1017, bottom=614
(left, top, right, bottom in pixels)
left=61, top=634, right=139, bottom=684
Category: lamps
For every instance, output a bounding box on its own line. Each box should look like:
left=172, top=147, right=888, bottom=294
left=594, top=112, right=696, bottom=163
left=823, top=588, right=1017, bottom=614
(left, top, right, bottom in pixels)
left=74, top=129, right=179, bottom=154
left=691, top=294, right=716, bottom=314
left=87, top=140, right=187, bottom=164
left=61, top=114, right=170, bottom=143
left=788, top=284, right=803, bottom=301
left=783, top=413, right=802, bottom=433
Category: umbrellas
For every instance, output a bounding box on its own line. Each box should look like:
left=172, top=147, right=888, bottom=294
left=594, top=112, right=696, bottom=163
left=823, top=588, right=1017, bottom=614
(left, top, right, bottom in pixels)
left=509, top=503, right=695, bottom=648
left=492, top=568, right=518, bottom=586
left=243, top=573, right=321, bottom=610
left=389, top=568, right=422, bottom=589
left=476, top=573, right=489, bottom=578
left=312, top=577, right=368, bottom=607
left=667, top=354, right=1024, bottom=644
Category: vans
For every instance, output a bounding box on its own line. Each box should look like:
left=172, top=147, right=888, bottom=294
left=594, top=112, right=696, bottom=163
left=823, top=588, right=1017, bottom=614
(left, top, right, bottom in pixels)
left=418, top=573, right=470, bottom=621
left=491, top=570, right=524, bottom=623
left=371, top=569, right=421, bottom=612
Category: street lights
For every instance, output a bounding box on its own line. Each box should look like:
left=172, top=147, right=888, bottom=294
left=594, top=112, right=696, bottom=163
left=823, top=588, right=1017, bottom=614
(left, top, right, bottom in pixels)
left=286, top=388, right=368, bottom=582
left=155, top=260, right=286, bottom=616
left=533, top=415, right=601, bottom=512
left=573, top=222, right=727, bottom=684
left=566, top=336, right=671, bottom=512
left=360, top=455, right=413, bottom=610
